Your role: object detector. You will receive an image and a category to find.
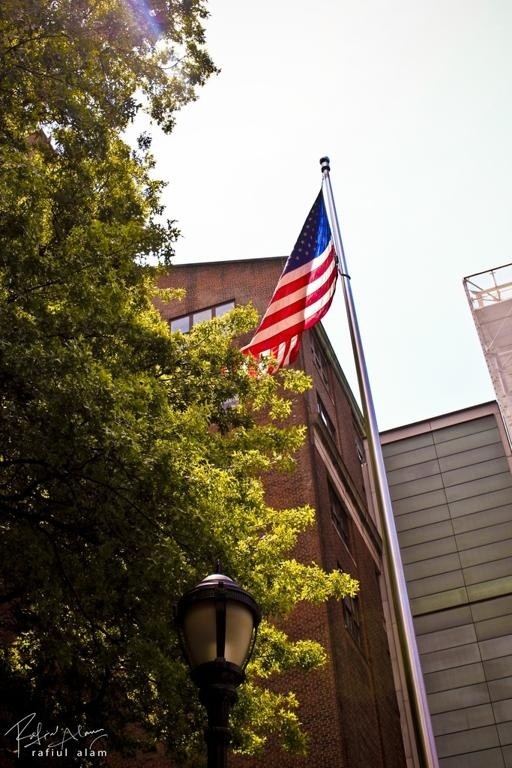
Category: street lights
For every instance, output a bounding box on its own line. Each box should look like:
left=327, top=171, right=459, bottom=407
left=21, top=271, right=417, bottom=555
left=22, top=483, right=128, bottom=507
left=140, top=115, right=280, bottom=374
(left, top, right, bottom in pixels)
left=177, top=571, right=260, bottom=768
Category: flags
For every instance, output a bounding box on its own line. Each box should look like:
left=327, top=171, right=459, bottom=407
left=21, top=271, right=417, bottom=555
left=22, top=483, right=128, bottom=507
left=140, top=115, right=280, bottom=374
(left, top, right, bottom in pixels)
left=217, top=181, right=340, bottom=383
